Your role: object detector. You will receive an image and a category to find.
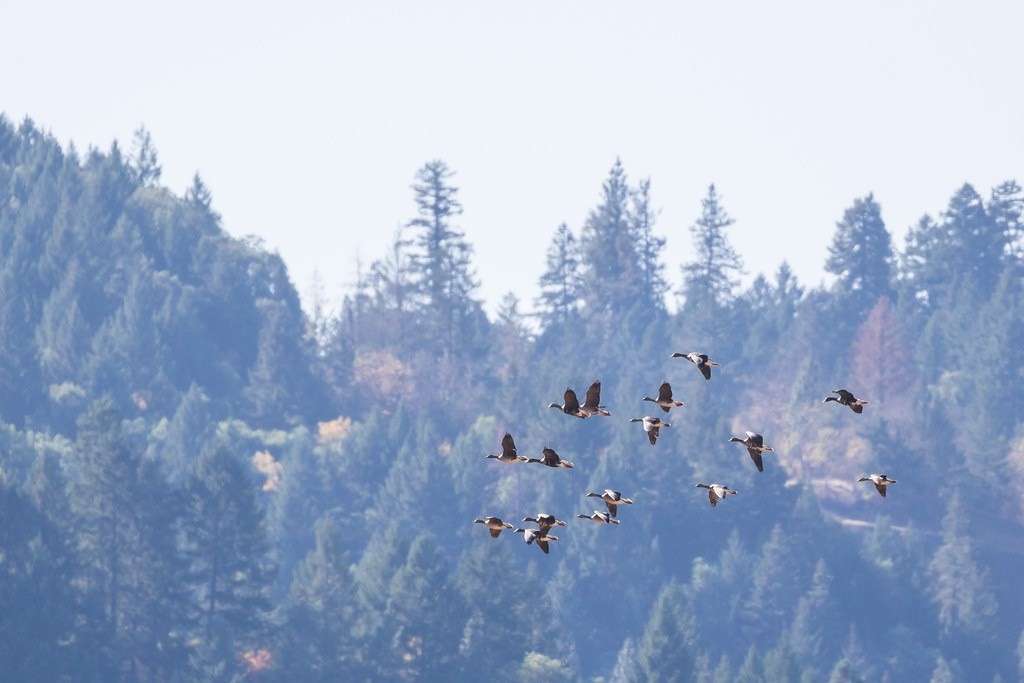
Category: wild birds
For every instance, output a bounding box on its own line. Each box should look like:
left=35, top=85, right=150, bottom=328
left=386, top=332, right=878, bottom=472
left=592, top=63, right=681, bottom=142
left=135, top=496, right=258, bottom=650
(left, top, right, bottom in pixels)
left=471, top=351, right=898, bottom=556
